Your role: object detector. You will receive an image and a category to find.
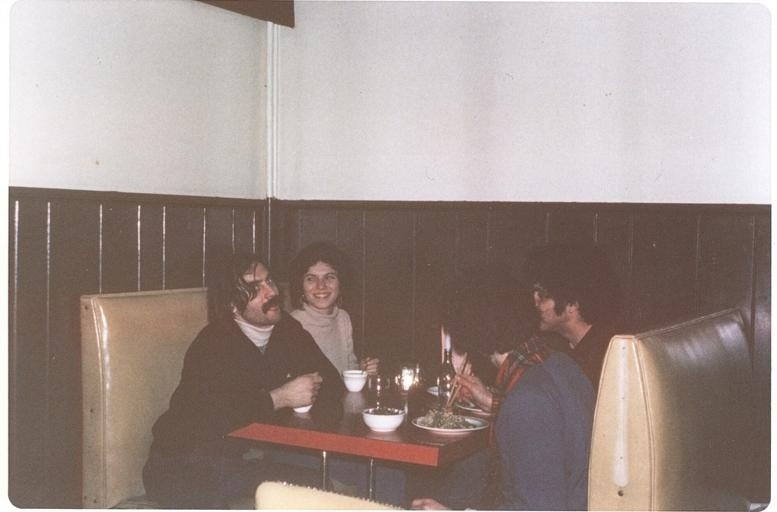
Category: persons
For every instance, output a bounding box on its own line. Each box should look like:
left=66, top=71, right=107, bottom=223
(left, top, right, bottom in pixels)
left=532, top=267, right=620, bottom=392
left=284, top=239, right=380, bottom=379
left=140, top=250, right=349, bottom=509
left=410, top=290, right=596, bottom=511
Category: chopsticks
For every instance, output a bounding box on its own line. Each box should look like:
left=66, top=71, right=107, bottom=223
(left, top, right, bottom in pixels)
left=445, top=352, right=472, bottom=408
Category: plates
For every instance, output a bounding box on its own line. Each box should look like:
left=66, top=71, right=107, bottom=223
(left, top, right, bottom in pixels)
left=411, top=413, right=490, bottom=436
left=454, top=402, right=496, bottom=416
left=426, top=386, right=453, bottom=397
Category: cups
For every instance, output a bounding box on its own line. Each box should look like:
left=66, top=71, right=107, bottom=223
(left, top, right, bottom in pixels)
left=395, top=367, right=414, bottom=393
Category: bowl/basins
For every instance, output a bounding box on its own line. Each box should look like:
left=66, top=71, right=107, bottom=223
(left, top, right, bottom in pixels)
left=342, top=370, right=368, bottom=392
left=361, top=407, right=405, bottom=432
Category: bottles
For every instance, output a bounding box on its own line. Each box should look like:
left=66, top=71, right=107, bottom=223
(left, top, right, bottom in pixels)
left=411, top=362, right=424, bottom=391
left=439, top=328, right=456, bottom=415
